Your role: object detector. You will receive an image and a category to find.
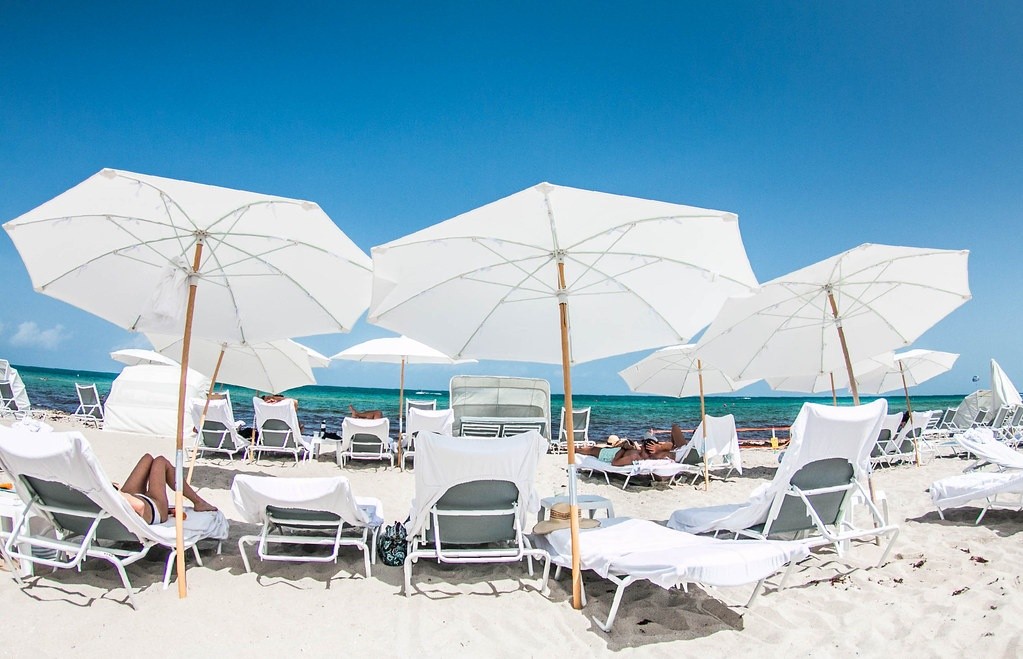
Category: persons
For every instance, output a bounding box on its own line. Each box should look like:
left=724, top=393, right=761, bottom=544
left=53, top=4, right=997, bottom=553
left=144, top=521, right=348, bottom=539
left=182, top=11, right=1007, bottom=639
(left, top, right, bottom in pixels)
left=265, top=394, right=304, bottom=432
left=670, top=423, right=698, bottom=448
left=113, top=454, right=218, bottom=524
left=893, top=411, right=908, bottom=440
left=574, top=438, right=674, bottom=467
left=349, top=405, right=382, bottom=419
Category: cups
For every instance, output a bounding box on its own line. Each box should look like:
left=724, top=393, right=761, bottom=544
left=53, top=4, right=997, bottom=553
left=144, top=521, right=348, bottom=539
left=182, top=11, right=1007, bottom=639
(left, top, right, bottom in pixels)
left=771, top=438, right=778, bottom=448
left=0, top=473, right=13, bottom=490
left=313, top=431, right=319, bottom=438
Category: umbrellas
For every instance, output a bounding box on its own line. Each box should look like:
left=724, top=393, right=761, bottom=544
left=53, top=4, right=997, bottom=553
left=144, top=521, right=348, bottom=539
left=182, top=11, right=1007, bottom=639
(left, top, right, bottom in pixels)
left=2, top=169, right=370, bottom=599
left=369, top=182, right=760, bottom=606
left=111, top=350, right=178, bottom=367
left=330, top=336, right=478, bottom=467
left=957, top=389, right=994, bottom=428
left=764, top=352, right=895, bottom=406
left=616, top=345, right=764, bottom=490
left=146, top=330, right=317, bottom=483
left=850, top=348, right=959, bottom=466
left=246, top=341, right=329, bottom=462
left=989, top=359, right=1022, bottom=451
left=692, top=240, right=973, bottom=544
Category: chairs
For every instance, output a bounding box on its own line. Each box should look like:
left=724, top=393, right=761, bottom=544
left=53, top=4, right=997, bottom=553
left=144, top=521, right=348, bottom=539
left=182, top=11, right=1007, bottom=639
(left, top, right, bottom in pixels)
left=0, top=357, right=1023, bottom=631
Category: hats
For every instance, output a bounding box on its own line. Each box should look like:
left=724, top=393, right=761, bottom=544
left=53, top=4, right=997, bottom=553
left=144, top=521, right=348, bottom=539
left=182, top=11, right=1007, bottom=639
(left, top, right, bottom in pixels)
left=532, top=503, right=601, bottom=536
left=594, top=435, right=619, bottom=448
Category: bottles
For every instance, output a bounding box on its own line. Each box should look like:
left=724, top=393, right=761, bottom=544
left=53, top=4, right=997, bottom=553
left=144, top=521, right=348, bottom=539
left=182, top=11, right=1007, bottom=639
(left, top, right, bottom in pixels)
left=321, top=420, right=326, bottom=439
